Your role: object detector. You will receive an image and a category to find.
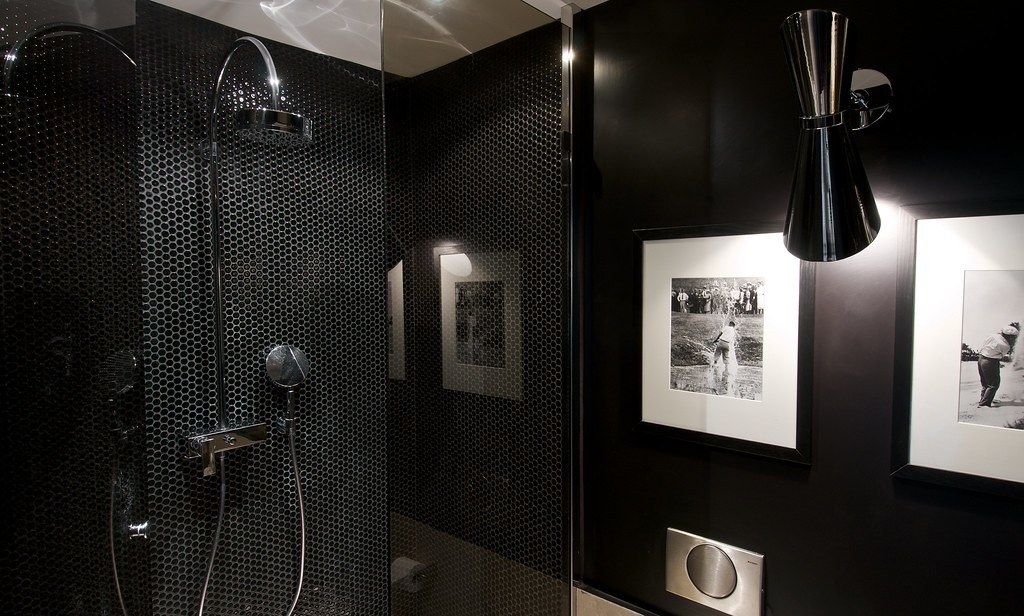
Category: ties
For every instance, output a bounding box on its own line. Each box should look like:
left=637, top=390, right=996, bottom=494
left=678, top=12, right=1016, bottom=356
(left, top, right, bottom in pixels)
left=682, top=292, right=683, bottom=300
left=704, top=291, right=705, bottom=296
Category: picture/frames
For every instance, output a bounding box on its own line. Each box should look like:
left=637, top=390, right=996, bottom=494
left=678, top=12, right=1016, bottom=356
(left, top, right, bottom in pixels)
left=430, top=239, right=535, bottom=419
left=634, top=219, right=815, bottom=467
left=888, top=196, right=1024, bottom=502
left=386, top=249, right=415, bottom=387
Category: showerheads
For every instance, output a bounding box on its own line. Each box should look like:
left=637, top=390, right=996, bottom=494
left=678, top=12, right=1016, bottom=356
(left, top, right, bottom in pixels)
left=1, top=91, right=41, bottom=129
left=236, top=107, right=314, bottom=147
left=99, top=349, right=142, bottom=438
left=265, top=344, right=310, bottom=435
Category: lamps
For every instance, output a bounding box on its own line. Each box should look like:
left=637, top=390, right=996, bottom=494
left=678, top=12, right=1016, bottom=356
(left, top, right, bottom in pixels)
left=782, top=6, right=895, bottom=265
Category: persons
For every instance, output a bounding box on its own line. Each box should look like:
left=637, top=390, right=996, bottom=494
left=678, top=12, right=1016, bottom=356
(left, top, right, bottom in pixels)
left=978, top=327, right=1018, bottom=408
left=711, top=321, right=735, bottom=374
left=675, top=281, right=764, bottom=315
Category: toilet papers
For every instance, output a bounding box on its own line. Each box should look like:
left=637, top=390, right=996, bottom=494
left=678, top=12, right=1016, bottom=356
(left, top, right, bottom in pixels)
left=390, top=556, right=427, bottom=594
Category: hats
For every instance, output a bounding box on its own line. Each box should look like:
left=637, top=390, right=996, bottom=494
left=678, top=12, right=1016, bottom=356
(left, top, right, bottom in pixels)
left=1002, top=326, right=1019, bottom=335
